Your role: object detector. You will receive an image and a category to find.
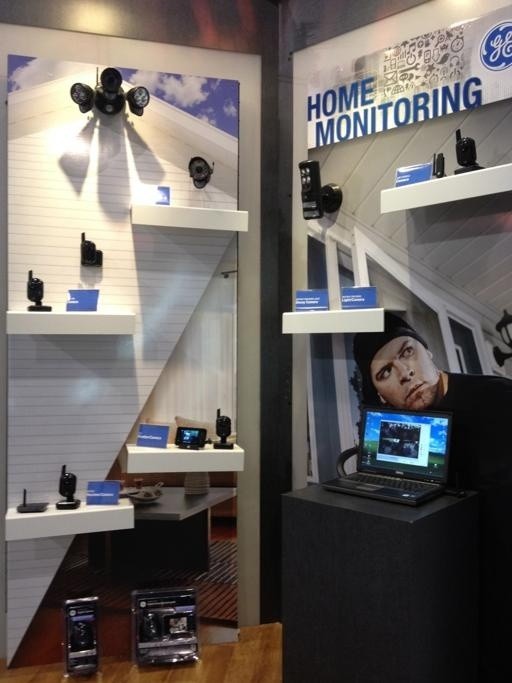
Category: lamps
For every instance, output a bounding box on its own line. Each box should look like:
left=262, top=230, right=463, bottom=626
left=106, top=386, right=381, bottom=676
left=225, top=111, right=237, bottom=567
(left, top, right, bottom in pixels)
left=70, top=67, right=150, bottom=116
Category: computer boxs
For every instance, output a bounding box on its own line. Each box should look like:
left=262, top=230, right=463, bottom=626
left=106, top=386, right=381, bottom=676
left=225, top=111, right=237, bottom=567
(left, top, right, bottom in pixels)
left=131, top=587, right=199, bottom=665
left=65, top=596, right=98, bottom=675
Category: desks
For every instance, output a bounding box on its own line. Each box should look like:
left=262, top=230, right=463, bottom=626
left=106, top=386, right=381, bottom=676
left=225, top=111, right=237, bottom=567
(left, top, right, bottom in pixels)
left=89, top=484, right=237, bottom=573
left=277, top=474, right=496, bottom=682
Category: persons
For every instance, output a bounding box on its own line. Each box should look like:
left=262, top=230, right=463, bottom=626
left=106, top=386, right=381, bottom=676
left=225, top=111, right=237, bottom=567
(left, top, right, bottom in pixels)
left=353, top=311, right=512, bottom=522
left=382, top=420, right=422, bottom=441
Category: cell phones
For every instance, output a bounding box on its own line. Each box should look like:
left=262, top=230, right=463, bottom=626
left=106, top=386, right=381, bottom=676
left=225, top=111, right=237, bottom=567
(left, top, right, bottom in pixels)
left=175, top=427, right=206, bottom=448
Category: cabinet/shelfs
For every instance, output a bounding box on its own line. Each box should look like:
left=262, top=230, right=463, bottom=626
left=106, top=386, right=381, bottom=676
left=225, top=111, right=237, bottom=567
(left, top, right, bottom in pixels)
left=6, top=203, right=249, bottom=544
left=280, top=0, right=511, bottom=487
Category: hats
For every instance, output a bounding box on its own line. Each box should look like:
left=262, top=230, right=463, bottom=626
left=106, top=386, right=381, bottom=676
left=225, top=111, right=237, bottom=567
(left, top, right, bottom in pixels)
left=352, top=311, right=428, bottom=387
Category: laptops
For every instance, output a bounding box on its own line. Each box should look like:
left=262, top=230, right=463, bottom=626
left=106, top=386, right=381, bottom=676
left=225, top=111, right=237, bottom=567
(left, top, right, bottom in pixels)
left=322, top=407, right=454, bottom=507
